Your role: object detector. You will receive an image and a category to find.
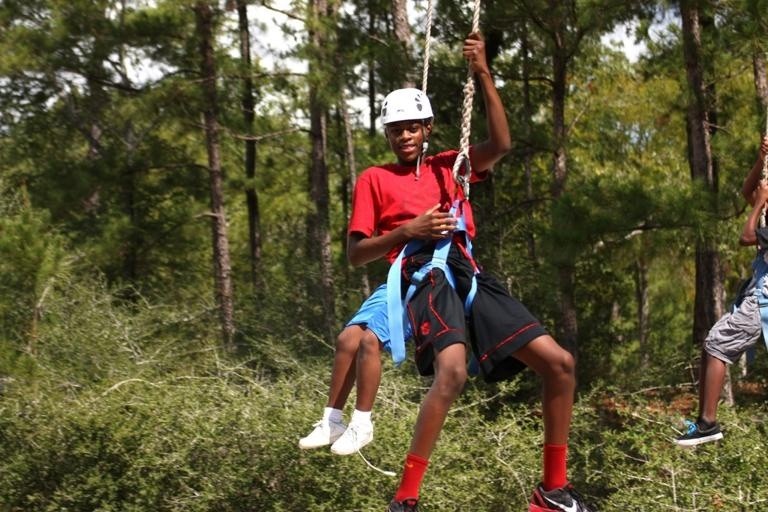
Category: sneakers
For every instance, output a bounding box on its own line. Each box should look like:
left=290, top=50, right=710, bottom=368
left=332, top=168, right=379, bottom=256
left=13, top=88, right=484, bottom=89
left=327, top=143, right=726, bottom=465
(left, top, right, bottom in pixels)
left=675, top=418, right=723, bottom=445
left=388, top=495, right=421, bottom=512
left=330, top=420, right=373, bottom=456
left=298, top=416, right=347, bottom=449
left=527, top=482, right=593, bottom=512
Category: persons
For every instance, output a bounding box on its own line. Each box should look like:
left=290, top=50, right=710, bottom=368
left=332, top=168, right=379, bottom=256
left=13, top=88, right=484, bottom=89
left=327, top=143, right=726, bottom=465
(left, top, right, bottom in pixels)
left=298, top=285, right=413, bottom=455
left=348, top=30, right=592, bottom=512
left=674, top=135, right=768, bottom=446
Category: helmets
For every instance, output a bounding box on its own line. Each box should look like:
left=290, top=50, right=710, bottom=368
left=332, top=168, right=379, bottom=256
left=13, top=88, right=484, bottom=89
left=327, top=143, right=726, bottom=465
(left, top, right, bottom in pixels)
left=380, top=88, right=434, bottom=125
